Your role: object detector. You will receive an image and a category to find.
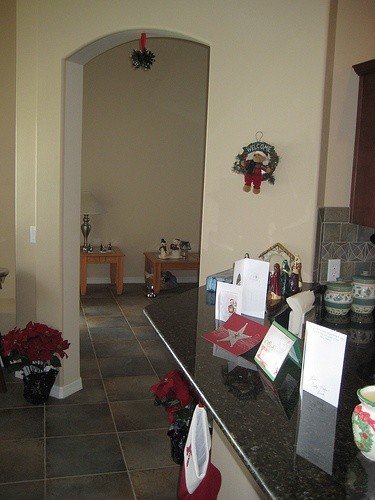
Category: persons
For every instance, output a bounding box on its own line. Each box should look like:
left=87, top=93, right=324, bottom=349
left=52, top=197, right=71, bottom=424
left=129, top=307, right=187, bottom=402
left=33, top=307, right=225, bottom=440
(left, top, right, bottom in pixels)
left=270, top=263, right=280, bottom=296
left=159, top=239, right=168, bottom=257
left=281, top=260, right=290, bottom=295
left=103, top=244, right=112, bottom=252
left=170, top=239, right=181, bottom=256
left=290, top=258, right=302, bottom=292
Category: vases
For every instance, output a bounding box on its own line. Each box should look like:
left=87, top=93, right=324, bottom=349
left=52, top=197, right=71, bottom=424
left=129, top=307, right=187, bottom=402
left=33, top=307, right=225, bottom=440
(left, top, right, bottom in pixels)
left=168, top=428, right=188, bottom=465
left=23, top=373, right=56, bottom=404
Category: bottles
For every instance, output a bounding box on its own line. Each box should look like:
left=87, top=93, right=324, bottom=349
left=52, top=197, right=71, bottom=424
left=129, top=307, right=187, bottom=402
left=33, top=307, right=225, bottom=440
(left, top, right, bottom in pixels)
left=325, top=312, right=375, bottom=348
left=322, top=277, right=354, bottom=316
left=350, top=270, right=375, bottom=314
left=351, top=385, right=375, bottom=461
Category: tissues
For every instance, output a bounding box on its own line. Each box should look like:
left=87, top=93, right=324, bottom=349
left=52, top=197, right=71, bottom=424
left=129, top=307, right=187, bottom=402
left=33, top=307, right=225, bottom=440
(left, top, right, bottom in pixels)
left=285, top=290, right=316, bottom=340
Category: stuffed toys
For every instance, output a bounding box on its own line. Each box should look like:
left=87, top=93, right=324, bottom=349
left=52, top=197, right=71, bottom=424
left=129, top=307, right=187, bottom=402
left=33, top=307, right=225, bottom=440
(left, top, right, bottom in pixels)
left=241, top=154, right=271, bottom=193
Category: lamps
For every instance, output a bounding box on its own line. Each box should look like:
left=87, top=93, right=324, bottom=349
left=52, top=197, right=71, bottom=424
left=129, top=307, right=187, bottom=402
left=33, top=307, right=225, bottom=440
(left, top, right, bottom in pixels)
left=80, top=192, right=105, bottom=252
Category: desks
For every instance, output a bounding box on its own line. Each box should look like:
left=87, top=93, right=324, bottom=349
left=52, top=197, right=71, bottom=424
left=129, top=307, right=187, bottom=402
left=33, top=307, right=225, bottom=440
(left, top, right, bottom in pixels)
left=144, top=251, right=199, bottom=296
left=80, top=246, right=126, bottom=295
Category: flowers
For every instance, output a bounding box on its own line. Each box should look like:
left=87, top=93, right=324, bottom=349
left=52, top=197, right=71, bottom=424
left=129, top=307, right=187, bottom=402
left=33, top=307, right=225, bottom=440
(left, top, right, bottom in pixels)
left=0, top=322, right=71, bottom=379
left=149, top=369, right=199, bottom=436
